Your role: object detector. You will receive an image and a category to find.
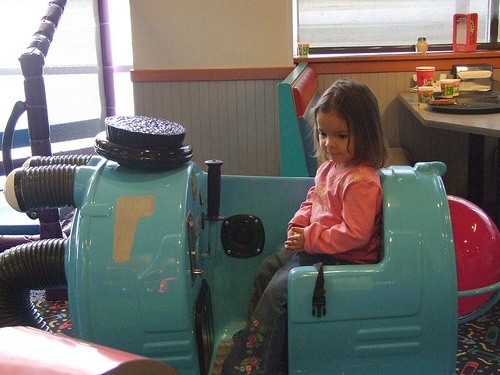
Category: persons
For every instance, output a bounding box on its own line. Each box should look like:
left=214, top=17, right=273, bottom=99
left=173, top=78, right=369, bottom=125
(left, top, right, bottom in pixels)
left=221, top=79, right=388, bottom=375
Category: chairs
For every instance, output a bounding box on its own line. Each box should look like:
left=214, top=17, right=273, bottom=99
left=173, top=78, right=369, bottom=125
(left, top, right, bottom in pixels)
left=277, top=63, right=324, bottom=178
left=287, top=161, right=463, bottom=375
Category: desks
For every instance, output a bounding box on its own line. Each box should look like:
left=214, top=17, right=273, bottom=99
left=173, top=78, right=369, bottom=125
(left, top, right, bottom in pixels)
left=398, top=79, right=500, bottom=208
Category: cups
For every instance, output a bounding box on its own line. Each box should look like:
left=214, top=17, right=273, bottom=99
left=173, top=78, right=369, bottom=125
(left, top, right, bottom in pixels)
left=441, top=80, right=455, bottom=98
left=416, top=66, right=435, bottom=87
left=418, top=87, right=433, bottom=106
left=448, top=79, right=462, bottom=97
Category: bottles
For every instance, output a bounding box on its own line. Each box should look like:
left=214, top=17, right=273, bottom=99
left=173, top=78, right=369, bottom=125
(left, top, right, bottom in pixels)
left=298, top=42, right=309, bottom=57
left=416, top=37, right=429, bottom=54
